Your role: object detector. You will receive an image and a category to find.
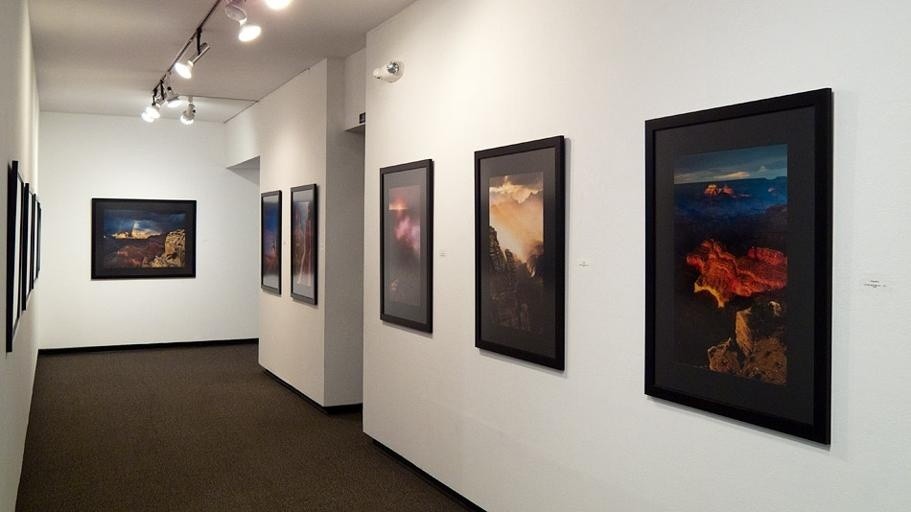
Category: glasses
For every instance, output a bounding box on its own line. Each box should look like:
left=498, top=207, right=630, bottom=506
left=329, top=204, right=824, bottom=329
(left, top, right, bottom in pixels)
left=141, top=0, right=295, bottom=126
left=371, top=61, right=405, bottom=82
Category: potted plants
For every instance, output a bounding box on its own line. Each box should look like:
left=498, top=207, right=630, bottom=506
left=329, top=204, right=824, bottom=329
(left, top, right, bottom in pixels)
left=256, top=190, right=281, bottom=296
left=379, top=159, right=434, bottom=335
left=475, top=136, right=567, bottom=370
left=645, top=88, right=832, bottom=444
left=91, top=198, right=197, bottom=279
left=291, top=184, right=319, bottom=305
left=11, top=162, right=43, bottom=350
